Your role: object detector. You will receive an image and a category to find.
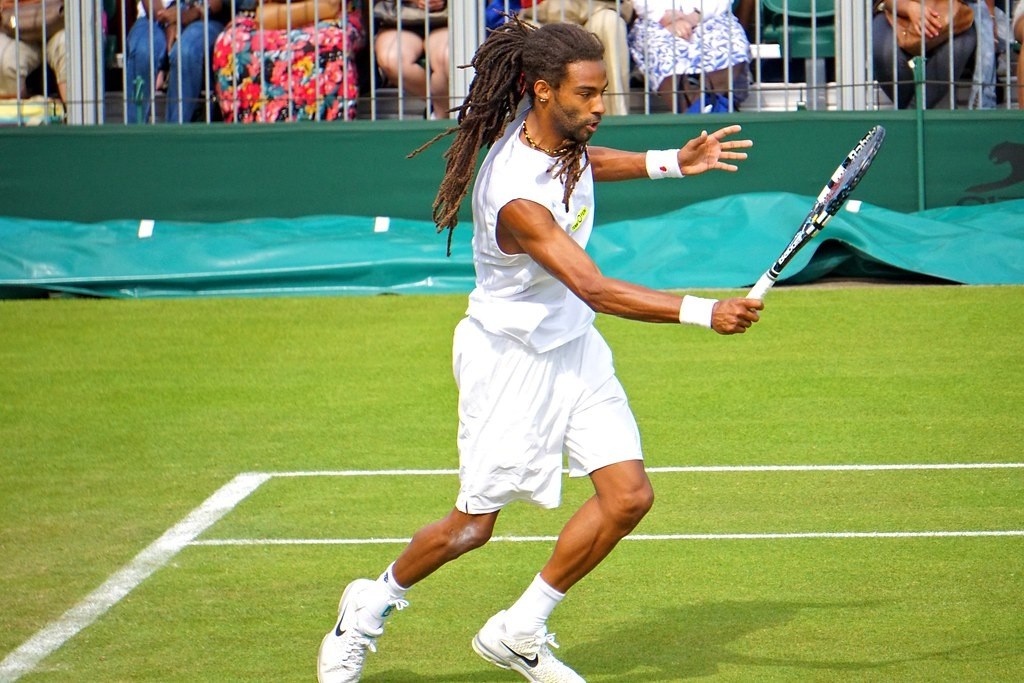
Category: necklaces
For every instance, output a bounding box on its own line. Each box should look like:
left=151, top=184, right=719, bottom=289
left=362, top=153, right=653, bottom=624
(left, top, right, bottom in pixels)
left=522, top=117, right=569, bottom=155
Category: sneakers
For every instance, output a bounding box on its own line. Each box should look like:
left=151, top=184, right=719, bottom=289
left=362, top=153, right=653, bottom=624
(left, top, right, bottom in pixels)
left=472, top=610, right=586, bottom=683
left=317, top=579, right=409, bottom=683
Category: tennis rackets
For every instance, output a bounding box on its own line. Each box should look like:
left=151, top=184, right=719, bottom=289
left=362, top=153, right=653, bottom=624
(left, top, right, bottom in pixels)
left=742, top=125, right=886, bottom=314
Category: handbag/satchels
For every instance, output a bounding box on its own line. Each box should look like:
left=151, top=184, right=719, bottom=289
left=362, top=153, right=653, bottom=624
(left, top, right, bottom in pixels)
left=0, top=0, right=64, bottom=42
left=883, top=0, right=974, bottom=56
left=254, top=0, right=340, bottom=29
left=680, top=65, right=734, bottom=113
left=374, top=0, right=448, bottom=25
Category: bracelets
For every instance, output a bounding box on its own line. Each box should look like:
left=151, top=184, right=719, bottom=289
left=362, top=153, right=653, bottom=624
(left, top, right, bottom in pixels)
left=645, top=148, right=685, bottom=180
left=694, top=8, right=700, bottom=14
left=678, top=294, right=720, bottom=330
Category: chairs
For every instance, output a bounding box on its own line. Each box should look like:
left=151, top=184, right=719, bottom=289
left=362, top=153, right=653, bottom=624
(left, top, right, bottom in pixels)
left=763, top=0, right=834, bottom=110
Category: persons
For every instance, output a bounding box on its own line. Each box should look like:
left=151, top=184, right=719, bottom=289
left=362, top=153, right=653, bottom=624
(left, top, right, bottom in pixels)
left=0, top=0, right=1024, bottom=125
left=316, top=8, right=764, bottom=683
